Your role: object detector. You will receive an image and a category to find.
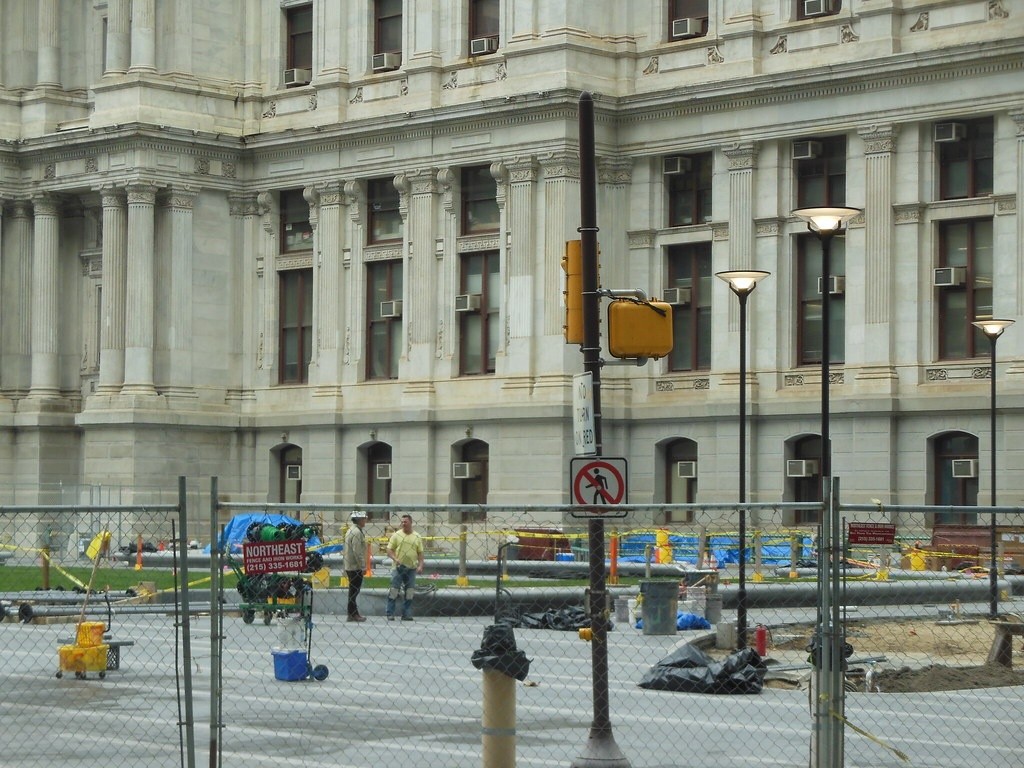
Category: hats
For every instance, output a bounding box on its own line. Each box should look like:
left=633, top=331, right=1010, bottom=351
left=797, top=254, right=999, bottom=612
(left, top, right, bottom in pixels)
left=351, top=511, right=368, bottom=517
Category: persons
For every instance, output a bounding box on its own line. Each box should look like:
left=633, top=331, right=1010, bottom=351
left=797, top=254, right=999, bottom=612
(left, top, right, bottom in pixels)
left=344, top=511, right=368, bottom=622
left=386, top=515, right=423, bottom=621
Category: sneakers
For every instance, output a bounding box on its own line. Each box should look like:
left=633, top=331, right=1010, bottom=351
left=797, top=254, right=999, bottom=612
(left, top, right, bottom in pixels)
left=387, top=614, right=394, bottom=621
left=347, top=613, right=365, bottom=622
left=401, top=615, right=414, bottom=621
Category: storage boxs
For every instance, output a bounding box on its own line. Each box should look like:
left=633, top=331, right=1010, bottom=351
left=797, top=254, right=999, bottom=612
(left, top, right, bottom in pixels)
left=272, top=647, right=310, bottom=681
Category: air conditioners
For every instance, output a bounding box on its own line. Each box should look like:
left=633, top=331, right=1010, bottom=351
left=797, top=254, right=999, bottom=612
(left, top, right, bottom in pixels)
left=805, top=0, right=833, bottom=15
left=934, top=268, right=966, bottom=285
left=793, top=141, right=821, bottom=158
left=283, top=68, right=311, bottom=84
left=935, top=123, right=966, bottom=141
left=663, top=289, right=691, bottom=304
left=952, top=460, right=979, bottom=477
left=471, top=38, right=498, bottom=54
left=787, top=460, right=818, bottom=477
left=287, top=465, right=302, bottom=480
left=373, top=53, right=401, bottom=69
left=380, top=301, right=401, bottom=317
left=818, top=276, right=845, bottom=294
left=455, top=295, right=481, bottom=311
left=664, top=157, right=693, bottom=173
left=377, top=464, right=391, bottom=479
left=678, top=461, right=697, bottom=478
left=453, top=462, right=481, bottom=478
left=673, top=18, right=703, bottom=37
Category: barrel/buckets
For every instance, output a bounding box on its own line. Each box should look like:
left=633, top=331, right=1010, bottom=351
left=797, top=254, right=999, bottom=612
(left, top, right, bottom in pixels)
left=614, top=587, right=722, bottom=624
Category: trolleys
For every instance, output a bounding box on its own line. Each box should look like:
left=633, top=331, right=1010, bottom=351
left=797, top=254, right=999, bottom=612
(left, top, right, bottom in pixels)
left=297, top=588, right=329, bottom=681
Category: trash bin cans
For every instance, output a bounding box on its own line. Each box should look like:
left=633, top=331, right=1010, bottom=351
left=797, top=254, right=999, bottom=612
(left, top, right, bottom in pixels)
left=639, top=570, right=680, bottom=635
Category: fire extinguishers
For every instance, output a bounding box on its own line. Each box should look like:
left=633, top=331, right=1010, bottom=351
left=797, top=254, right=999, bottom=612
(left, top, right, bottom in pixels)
left=755, top=623, right=766, bottom=656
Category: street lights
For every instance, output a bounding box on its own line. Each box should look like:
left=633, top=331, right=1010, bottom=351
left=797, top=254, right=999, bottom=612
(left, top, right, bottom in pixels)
left=972, top=318, right=1016, bottom=616
left=790, top=206, right=862, bottom=621
left=717, top=270, right=771, bottom=648
